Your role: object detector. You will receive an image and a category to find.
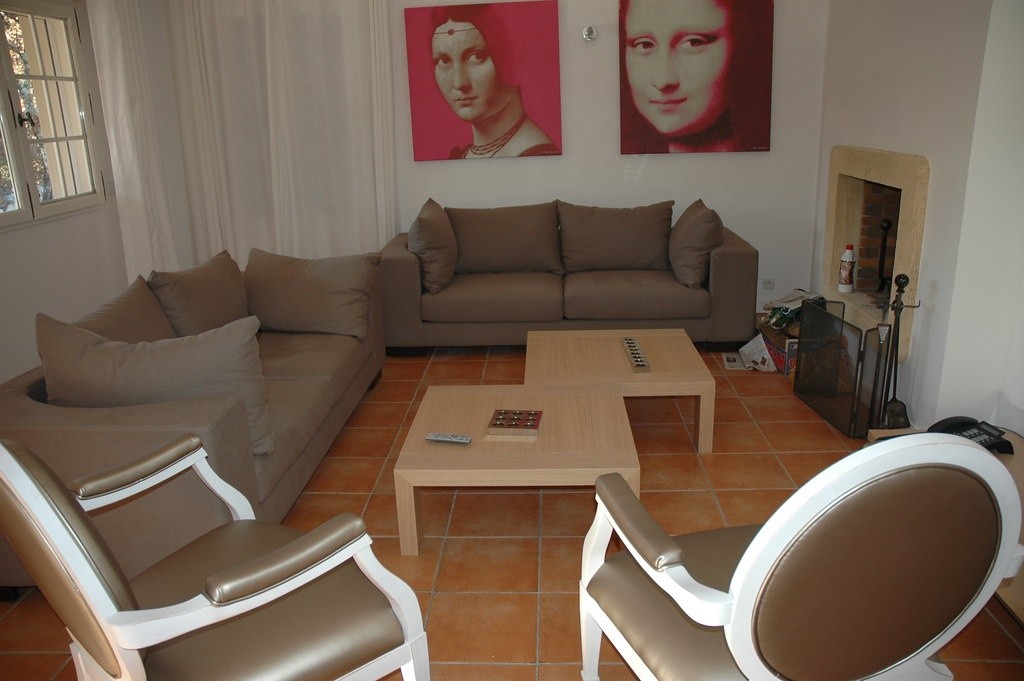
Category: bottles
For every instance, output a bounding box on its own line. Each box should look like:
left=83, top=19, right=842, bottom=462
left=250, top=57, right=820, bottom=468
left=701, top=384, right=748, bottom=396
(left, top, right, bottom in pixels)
left=838, top=245, right=855, bottom=292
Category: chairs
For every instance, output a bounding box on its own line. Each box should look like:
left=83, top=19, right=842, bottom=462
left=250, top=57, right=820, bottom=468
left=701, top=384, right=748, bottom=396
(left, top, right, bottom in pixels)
left=0, top=433, right=431, bottom=681
left=579, top=433, right=1024, bottom=681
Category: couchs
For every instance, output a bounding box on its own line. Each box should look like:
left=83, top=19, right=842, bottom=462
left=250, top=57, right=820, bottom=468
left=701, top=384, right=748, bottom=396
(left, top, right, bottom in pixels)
left=0, top=248, right=385, bottom=525
left=383, top=198, right=758, bottom=345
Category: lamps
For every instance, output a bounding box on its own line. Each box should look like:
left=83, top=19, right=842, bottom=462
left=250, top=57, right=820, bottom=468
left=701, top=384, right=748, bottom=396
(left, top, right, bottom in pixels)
left=581, top=28, right=598, bottom=45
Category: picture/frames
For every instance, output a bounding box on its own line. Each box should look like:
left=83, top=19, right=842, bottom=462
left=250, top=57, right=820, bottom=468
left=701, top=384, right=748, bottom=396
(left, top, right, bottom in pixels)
left=619, top=0, right=775, bottom=155
left=404, top=0, right=562, bottom=161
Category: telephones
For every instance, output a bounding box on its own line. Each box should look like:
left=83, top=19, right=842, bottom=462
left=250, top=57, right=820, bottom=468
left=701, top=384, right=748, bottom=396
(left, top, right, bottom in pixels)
left=927, top=416, right=1014, bottom=455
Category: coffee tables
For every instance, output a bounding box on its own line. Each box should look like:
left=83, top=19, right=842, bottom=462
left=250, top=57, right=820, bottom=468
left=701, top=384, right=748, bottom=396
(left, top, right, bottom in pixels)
left=393, top=329, right=716, bottom=557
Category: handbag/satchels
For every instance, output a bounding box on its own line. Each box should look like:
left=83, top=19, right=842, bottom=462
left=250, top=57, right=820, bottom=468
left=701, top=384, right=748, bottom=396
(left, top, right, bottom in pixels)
left=761, top=288, right=824, bottom=329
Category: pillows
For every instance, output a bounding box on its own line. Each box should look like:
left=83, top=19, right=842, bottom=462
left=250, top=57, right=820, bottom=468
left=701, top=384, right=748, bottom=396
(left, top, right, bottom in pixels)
left=408, top=200, right=723, bottom=290
left=38, top=250, right=371, bottom=452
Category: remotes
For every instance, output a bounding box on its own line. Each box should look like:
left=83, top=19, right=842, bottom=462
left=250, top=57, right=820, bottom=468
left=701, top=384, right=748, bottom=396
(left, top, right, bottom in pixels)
left=425, top=432, right=472, bottom=444
